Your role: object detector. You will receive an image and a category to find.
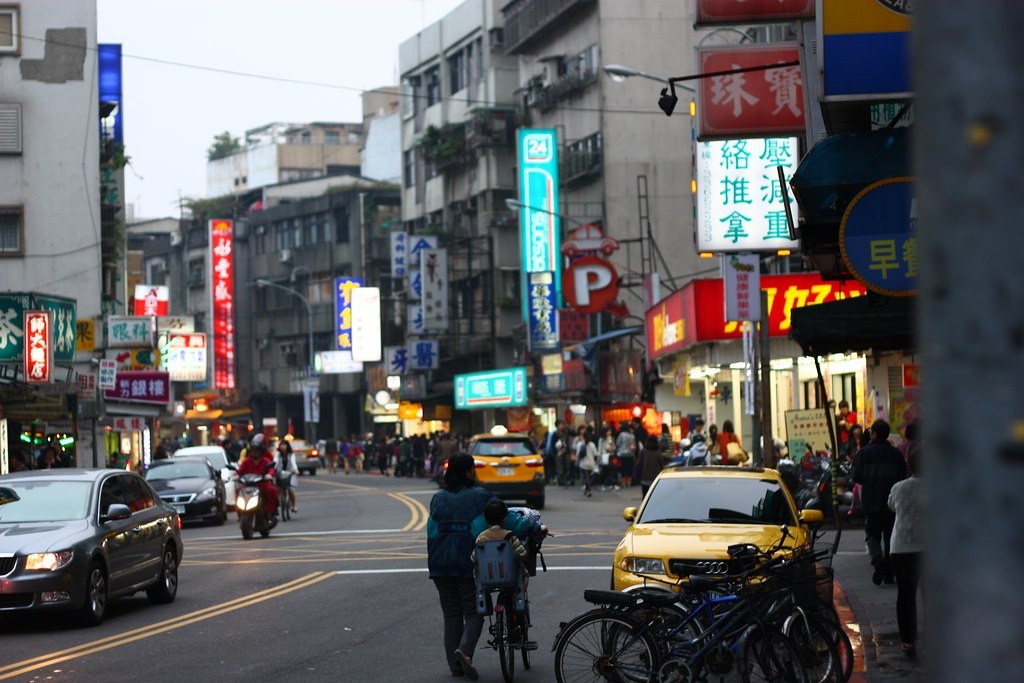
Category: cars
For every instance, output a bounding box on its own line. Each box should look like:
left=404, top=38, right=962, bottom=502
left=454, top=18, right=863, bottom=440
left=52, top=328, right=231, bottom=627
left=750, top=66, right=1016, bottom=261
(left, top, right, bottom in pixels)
left=146, top=457, right=228, bottom=527
left=467, top=425, right=546, bottom=509
left=609, top=466, right=825, bottom=592
left=268, top=433, right=319, bottom=476
left=0, top=468, right=184, bottom=630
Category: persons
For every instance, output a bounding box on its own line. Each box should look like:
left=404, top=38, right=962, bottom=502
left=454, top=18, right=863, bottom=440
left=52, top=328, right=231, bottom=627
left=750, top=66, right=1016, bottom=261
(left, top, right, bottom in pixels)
left=527, top=417, right=740, bottom=501
left=773, top=400, right=927, bottom=653
left=209, top=433, right=299, bottom=522
left=105, top=450, right=146, bottom=476
left=317, top=430, right=472, bottom=481
left=426, top=452, right=548, bottom=678
left=470, top=497, right=530, bottom=607
left=8, top=434, right=71, bottom=472
left=153, top=436, right=195, bottom=460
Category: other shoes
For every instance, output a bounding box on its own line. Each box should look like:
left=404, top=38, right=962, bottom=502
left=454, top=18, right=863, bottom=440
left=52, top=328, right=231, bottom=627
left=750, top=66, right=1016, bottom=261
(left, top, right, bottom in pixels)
left=901, top=642, right=915, bottom=654
left=584, top=490, right=591, bottom=497
left=293, top=507, right=297, bottom=512
left=451, top=669, right=463, bottom=677
left=883, top=577, right=894, bottom=585
left=873, top=558, right=885, bottom=585
left=454, top=649, right=478, bottom=679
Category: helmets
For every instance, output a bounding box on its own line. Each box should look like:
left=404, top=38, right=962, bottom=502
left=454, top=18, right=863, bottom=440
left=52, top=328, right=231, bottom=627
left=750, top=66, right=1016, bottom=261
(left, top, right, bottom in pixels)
left=253, top=433, right=266, bottom=445
left=250, top=441, right=265, bottom=452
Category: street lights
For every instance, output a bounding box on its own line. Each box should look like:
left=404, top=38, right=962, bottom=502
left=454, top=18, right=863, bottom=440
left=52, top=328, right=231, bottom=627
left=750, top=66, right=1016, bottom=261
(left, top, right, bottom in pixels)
left=600, top=64, right=762, bottom=469
left=255, top=278, right=317, bottom=450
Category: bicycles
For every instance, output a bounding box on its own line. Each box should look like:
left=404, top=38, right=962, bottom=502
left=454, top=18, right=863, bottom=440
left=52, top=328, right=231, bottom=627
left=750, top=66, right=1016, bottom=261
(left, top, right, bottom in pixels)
left=550, top=523, right=855, bottom=683
left=274, top=468, right=297, bottom=523
left=480, top=526, right=556, bottom=683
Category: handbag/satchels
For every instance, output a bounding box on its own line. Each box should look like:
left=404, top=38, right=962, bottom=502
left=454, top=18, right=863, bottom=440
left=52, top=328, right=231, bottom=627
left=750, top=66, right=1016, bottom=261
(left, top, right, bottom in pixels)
left=578, top=443, right=586, bottom=459
left=693, top=458, right=707, bottom=468
left=726, top=432, right=747, bottom=464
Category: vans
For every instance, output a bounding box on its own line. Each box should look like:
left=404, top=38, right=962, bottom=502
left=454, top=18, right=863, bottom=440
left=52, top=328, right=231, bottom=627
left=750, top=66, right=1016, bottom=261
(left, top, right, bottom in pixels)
left=174, top=444, right=238, bottom=513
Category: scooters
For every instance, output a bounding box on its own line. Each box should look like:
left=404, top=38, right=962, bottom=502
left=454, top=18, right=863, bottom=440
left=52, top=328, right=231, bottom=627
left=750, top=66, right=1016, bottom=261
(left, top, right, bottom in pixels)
left=778, top=441, right=868, bottom=531
left=224, top=461, right=277, bottom=539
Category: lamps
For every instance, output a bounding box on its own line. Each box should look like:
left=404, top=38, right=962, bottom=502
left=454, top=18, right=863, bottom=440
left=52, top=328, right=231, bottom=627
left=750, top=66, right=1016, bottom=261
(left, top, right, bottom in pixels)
left=656, top=61, right=799, bottom=115
